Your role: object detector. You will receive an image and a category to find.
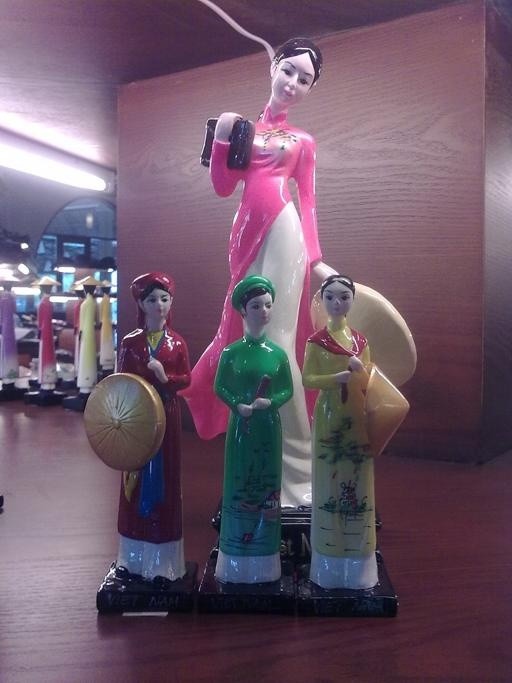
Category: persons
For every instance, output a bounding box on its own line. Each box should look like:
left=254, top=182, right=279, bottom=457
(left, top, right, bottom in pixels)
left=111, top=273, right=190, bottom=586
left=1, top=275, right=115, bottom=396
left=212, top=276, right=294, bottom=590
left=0, top=35, right=420, bottom=618
left=302, top=275, right=379, bottom=596
left=177, top=38, right=340, bottom=509
